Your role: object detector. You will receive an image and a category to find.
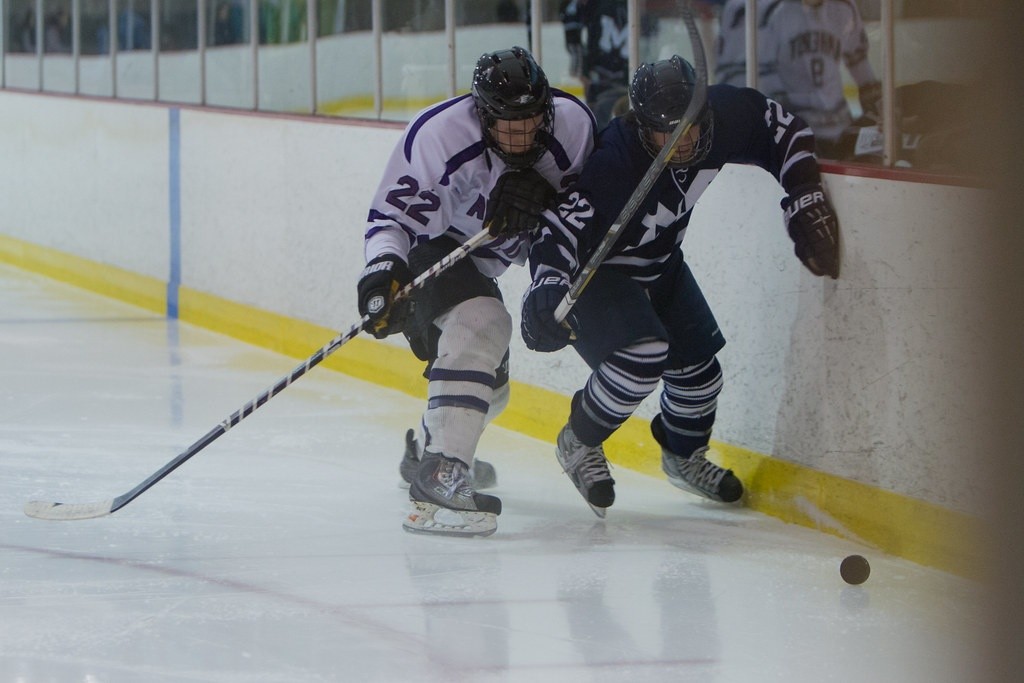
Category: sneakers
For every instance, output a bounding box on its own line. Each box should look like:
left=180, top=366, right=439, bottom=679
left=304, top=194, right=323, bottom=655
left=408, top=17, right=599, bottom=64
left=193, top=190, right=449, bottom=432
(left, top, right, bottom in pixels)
left=401, top=450, right=502, bottom=538
left=662, top=446, right=744, bottom=504
left=555, top=415, right=617, bottom=518
left=398, top=428, right=500, bottom=493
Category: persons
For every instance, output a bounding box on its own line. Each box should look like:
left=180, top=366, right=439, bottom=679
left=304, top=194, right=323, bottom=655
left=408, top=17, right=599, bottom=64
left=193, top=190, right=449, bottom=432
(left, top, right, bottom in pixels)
left=356, top=46, right=600, bottom=539
left=562, top=0, right=883, bottom=161
left=521, top=55, right=840, bottom=520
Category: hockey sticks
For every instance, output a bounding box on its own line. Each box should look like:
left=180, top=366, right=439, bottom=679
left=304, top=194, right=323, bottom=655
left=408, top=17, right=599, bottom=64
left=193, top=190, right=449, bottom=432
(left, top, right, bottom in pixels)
left=21, top=215, right=508, bottom=522
left=552, top=0, right=709, bottom=326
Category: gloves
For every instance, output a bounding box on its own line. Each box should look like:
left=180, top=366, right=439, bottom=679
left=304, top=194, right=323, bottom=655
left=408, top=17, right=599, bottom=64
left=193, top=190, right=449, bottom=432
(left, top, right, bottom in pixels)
left=357, top=254, right=410, bottom=338
left=482, top=167, right=544, bottom=237
left=780, top=184, right=840, bottom=278
left=519, top=271, right=581, bottom=352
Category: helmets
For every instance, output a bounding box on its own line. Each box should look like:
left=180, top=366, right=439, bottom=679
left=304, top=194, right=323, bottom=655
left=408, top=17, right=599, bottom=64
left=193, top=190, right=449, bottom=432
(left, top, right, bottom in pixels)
left=472, top=46, right=556, bottom=169
left=629, top=55, right=707, bottom=169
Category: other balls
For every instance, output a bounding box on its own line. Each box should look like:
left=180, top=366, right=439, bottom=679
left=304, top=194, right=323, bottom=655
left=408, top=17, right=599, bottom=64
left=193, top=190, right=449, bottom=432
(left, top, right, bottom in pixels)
left=839, top=554, right=871, bottom=586
